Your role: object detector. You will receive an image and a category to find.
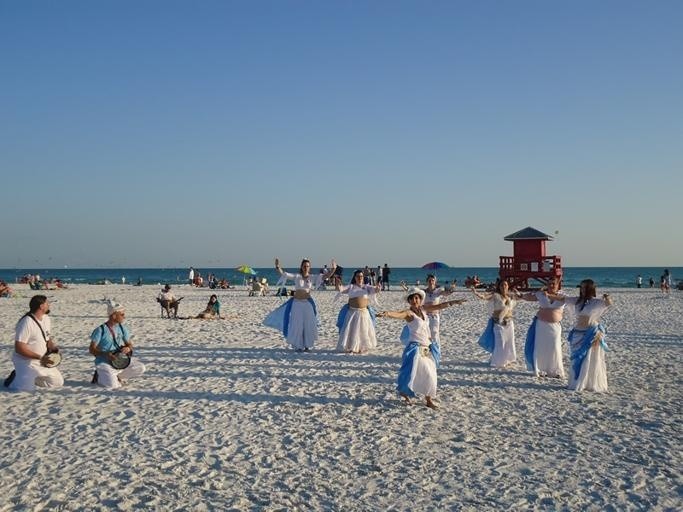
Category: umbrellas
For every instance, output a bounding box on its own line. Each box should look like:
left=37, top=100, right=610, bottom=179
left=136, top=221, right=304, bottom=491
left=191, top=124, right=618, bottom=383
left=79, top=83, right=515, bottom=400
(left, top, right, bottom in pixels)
left=422, top=263, right=449, bottom=275
left=234, top=264, right=256, bottom=280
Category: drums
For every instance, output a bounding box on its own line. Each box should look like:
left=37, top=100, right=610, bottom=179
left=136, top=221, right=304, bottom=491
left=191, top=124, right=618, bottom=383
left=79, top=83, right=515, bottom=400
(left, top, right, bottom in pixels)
left=44, top=352, right=62, bottom=368
left=111, top=351, right=131, bottom=368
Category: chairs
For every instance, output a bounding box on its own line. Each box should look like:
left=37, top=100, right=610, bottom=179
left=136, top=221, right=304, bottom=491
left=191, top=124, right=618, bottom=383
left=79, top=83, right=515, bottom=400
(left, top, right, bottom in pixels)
left=157, top=298, right=174, bottom=318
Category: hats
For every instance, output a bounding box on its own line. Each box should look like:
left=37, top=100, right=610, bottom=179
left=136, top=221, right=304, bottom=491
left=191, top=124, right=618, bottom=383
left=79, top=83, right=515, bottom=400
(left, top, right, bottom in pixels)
left=108, top=302, right=124, bottom=316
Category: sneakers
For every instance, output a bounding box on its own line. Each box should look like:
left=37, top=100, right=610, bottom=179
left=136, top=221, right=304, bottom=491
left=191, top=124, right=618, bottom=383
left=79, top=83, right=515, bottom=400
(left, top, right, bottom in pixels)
left=4, top=370, right=15, bottom=387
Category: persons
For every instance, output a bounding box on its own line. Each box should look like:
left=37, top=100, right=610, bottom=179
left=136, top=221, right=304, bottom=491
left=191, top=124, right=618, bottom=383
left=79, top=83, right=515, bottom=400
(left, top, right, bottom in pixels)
left=189, top=267, right=229, bottom=289
left=248, top=276, right=268, bottom=296
left=336, top=270, right=381, bottom=354
left=376, top=278, right=468, bottom=410
left=322, top=263, right=343, bottom=286
left=364, top=264, right=391, bottom=291
left=637, top=269, right=683, bottom=293
left=89, top=302, right=145, bottom=388
left=0, top=280, right=12, bottom=297
left=465, top=275, right=613, bottom=391
left=189, top=295, right=225, bottom=319
left=263, top=258, right=338, bottom=352
left=22, top=273, right=63, bottom=290
left=4, top=295, right=63, bottom=390
left=159, top=284, right=179, bottom=318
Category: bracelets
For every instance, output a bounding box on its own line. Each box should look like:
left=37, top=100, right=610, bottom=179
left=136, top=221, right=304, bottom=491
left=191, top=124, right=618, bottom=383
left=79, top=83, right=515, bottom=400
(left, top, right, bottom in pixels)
left=276, top=266, right=280, bottom=270
